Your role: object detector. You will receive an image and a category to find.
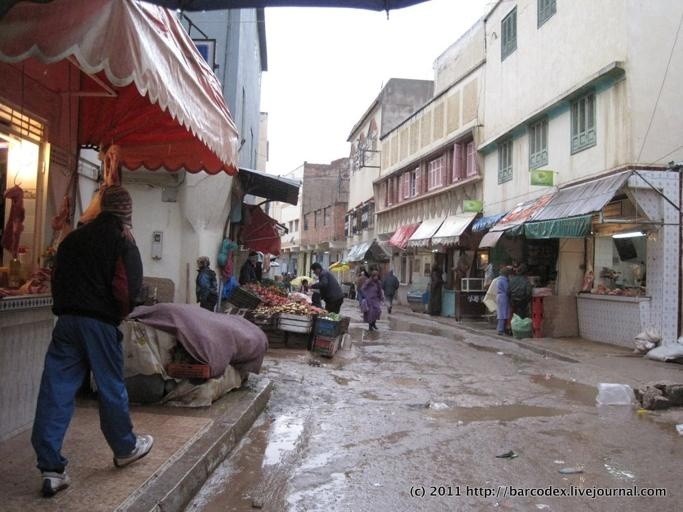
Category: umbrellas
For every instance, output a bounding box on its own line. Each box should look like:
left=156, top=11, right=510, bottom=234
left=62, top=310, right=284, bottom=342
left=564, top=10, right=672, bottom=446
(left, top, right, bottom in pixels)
left=289, top=275, right=314, bottom=288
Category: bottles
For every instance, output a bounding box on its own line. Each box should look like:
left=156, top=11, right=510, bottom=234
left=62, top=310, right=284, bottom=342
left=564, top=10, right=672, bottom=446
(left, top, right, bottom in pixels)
left=8, top=255, right=20, bottom=288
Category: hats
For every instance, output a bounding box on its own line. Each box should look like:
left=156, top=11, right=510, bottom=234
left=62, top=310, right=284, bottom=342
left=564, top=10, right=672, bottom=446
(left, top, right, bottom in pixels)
left=248, top=252, right=258, bottom=261
left=100, top=185, right=132, bottom=226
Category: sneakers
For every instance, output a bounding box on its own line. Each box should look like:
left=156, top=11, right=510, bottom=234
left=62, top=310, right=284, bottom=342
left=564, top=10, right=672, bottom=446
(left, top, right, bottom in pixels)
left=41, top=471, right=71, bottom=497
left=113, top=434, right=153, bottom=468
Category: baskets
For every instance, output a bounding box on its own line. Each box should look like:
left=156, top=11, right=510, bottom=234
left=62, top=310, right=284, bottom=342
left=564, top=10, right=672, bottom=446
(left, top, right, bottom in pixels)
left=168, top=364, right=211, bottom=379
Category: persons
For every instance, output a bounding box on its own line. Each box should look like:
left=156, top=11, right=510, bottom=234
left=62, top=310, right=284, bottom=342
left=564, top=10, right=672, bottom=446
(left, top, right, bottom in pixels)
left=298, top=279, right=311, bottom=294
left=30, top=184, right=154, bottom=498
left=194, top=256, right=218, bottom=312
left=306, top=262, right=344, bottom=314
left=239, top=251, right=258, bottom=285
left=354, top=262, right=399, bottom=335
left=425, top=261, right=444, bottom=317
left=280, top=271, right=295, bottom=291
left=494, top=261, right=531, bottom=337
